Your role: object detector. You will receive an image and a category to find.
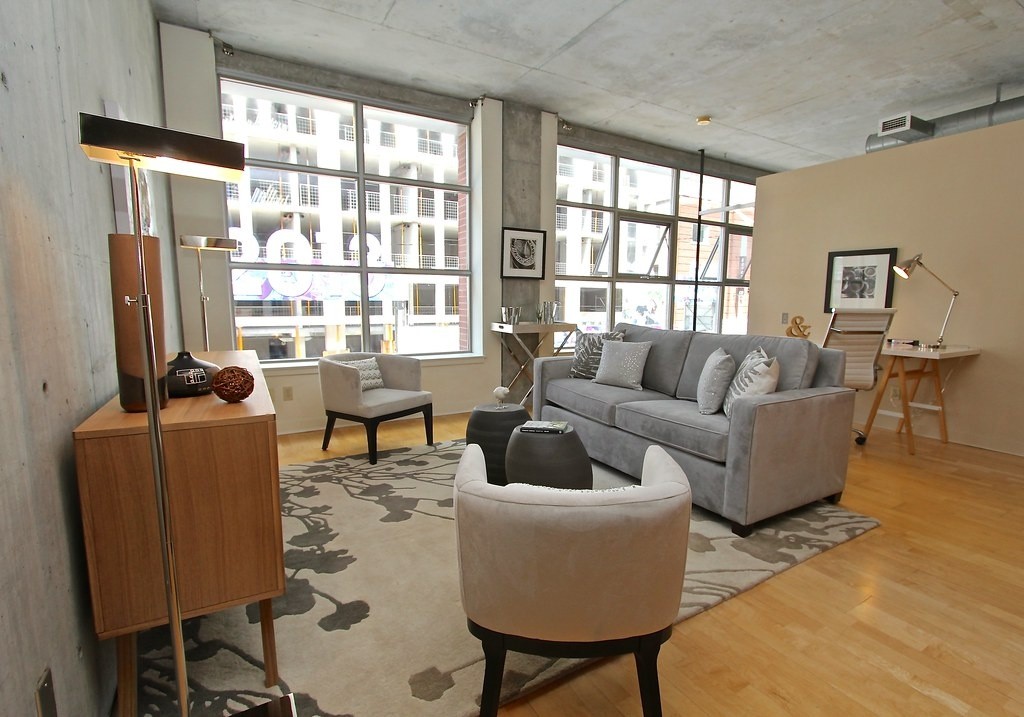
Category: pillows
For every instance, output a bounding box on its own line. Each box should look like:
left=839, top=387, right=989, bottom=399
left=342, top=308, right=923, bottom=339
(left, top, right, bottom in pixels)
left=724, top=347, right=781, bottom=420
left=567, top=327, right=627, bottom=379
left=592, top=340, right=653, bottom=391
left=697, top=346, right=737, bottom=415
left=333, top=357, right=384, bottom=391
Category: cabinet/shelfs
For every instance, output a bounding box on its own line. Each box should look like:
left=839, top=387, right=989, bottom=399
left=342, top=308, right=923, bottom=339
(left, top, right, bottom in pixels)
left=74, top=350, right=288, bottom=714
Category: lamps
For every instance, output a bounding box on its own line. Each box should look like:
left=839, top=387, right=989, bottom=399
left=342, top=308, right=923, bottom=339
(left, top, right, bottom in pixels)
left=180, top=236, right=238, bottom=362
left=78, top=113, right=247, bottom=717
left=892, top=254, right=958, bottom=350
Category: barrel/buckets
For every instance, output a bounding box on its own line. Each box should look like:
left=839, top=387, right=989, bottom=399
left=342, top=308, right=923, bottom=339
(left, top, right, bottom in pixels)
left=501, top=306, right=522, bottom=326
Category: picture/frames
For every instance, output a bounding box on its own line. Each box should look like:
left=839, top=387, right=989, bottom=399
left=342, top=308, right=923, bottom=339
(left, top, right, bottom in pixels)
left=823, top=248, right=898, bottom=313
left=500, top=227, right=548, bottom=279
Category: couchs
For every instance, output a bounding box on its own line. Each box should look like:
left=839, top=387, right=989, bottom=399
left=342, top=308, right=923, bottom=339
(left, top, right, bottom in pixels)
left=533, top=321, right=855, bottom=535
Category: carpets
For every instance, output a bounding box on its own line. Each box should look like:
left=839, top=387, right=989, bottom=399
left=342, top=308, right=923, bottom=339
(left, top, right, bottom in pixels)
left=103, top=438, right=879, bottom=716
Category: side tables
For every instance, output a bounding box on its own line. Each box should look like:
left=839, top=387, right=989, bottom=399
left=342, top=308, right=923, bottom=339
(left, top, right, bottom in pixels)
left=491, top=321, right=577, bottom=406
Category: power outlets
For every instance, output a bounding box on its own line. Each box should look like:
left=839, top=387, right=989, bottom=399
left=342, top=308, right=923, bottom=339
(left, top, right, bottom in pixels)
left=33, top=666, right=58, bottom=717
left=781, top=313, right=789, bottom=324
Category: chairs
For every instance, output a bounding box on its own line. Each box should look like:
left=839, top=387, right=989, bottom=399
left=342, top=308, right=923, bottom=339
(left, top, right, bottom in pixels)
left=317, top=352, right=434, bottom=465
left=451, top=443, right=692, bottom=717
left=820, top=306, right=898, bottom=444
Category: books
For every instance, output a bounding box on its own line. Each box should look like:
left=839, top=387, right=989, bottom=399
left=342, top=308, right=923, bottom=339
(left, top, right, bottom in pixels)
left=520, top=421, right=569, bottom=434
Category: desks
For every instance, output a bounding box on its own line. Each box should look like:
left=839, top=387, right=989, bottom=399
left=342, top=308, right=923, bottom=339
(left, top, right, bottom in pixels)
left=860, top=346, right=979, bottom=455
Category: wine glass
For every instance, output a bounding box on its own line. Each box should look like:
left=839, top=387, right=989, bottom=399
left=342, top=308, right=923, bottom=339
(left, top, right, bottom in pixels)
left=535, top=300, right=559, bottom=325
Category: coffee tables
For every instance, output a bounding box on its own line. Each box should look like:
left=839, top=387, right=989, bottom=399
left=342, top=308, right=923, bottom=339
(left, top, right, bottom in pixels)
left=505, top=421, right=593, bottom=488
left=465, top=401, right=531, bottom=488
